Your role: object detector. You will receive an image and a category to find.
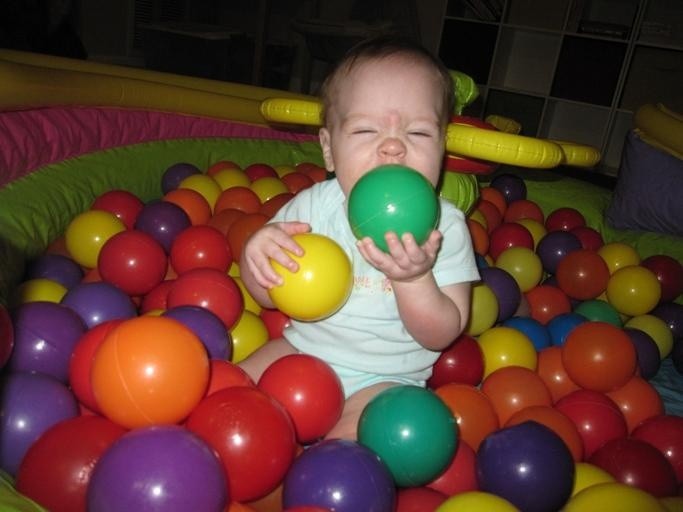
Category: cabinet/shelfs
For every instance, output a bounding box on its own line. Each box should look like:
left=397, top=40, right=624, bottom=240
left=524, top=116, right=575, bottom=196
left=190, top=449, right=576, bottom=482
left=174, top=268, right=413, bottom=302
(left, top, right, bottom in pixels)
left=321, top=1, right=683, bottom=173
left=76, top=0, right=321, bottom=96
